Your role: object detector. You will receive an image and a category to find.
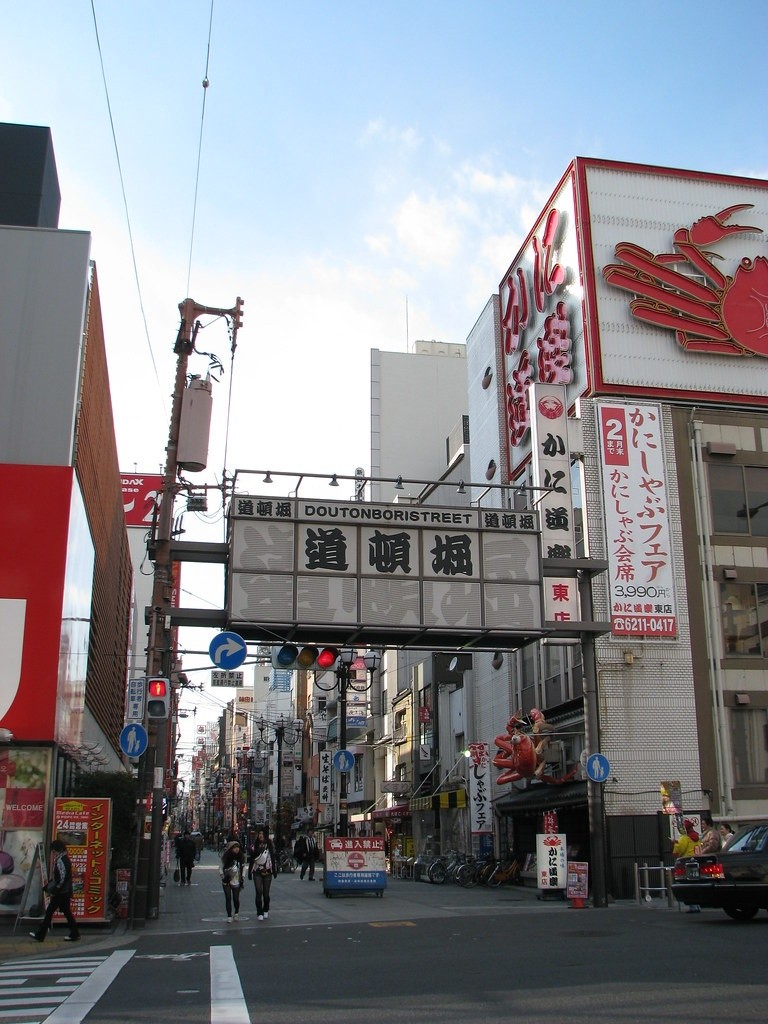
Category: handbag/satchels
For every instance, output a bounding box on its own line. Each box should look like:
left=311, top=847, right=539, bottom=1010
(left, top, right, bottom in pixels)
left=174, top=869, right=180, bottom=882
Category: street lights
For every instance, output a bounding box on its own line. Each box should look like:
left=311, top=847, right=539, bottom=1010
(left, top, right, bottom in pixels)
left=315, top=644, right=382, bottom=837
left=234, top=747, right=269, bottom=854
left=182, top=767, right=238, bottom=841
left=255, top=714, right=305, bottom=851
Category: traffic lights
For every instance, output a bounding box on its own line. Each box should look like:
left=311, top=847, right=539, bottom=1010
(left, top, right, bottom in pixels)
left=271, top=645, right=340, bottom=670
left=147, top=677, right=173, bottom=721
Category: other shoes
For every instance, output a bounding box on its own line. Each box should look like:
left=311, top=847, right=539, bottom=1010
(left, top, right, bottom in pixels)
left=29, top=932, right=45, bottom=942
left=227, top=914, right=239, bottom=923
left=64, top=934, right=81, bottom=941
left=180, top=880, right=192, bottom=888
left=308, top=877, right=315, bottom=881
left=300, top=874, right=304, bottom=880
left=258, top=912, right=269, bottom=921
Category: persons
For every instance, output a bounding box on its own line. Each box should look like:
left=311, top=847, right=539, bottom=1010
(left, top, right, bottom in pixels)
left=667, top=818, right=735, bottom=914
left=173, top=831, right=204, bottom=886
left=28, top=839, right=81, bottom=942
left=219, top=840, right=246, bottom=924
left=272, top=832, right=292, bottom=872
left=294, top=830, right=320, bottom=881
left=248, top=829, right=278, bottom=920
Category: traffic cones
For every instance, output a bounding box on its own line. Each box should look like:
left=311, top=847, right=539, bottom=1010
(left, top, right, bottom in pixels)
left=569, top=897, right=590, bottom=908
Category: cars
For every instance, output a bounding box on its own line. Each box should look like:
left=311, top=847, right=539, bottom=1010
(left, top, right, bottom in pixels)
left=190, top=831, right=204, bottom=847
left=674, top=822, right=768, bottom=919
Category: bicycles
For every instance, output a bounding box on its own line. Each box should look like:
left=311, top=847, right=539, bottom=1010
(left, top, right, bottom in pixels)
left=274, top=852, right=298, bottom=871
left=427, top=848, right=525, bottom=887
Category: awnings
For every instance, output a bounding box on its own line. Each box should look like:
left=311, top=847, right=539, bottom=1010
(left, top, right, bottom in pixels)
left=409, top=789, right=466, bottom=811
left=372, top=804, right=411, bottom=818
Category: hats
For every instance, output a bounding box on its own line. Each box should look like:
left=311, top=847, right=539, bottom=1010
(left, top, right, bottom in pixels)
left=225, top=841, right=239, bottom=852
